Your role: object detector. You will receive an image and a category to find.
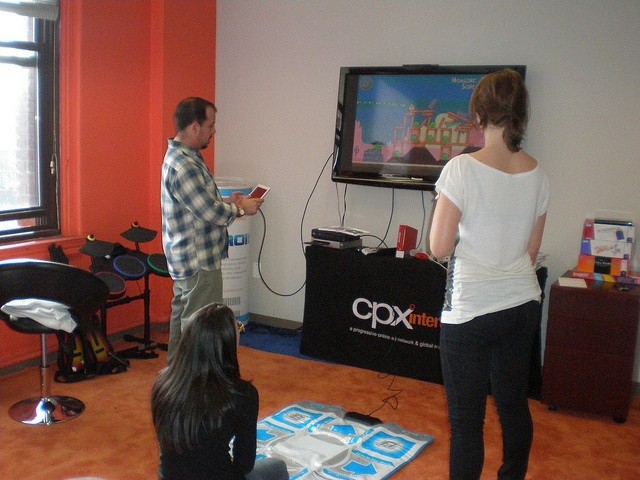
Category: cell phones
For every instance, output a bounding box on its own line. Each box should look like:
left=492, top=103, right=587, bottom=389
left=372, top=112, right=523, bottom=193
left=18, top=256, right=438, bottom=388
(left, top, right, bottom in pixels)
left=614, top=281, right=631, bottom=292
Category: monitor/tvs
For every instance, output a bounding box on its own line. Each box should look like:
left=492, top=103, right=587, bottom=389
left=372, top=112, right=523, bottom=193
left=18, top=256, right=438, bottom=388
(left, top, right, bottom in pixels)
left=331, top=66, right=525, bottom=190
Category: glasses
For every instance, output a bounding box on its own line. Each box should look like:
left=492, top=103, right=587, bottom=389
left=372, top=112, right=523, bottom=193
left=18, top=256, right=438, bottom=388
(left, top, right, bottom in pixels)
left=238, top=320, right=245, bottom=336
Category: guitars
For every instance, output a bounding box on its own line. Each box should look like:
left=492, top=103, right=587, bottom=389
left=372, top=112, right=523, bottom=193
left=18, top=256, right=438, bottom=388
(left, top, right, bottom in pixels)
left=47, top=243, right=89, bottom=381
left=57, top=246, right=130, bottom=375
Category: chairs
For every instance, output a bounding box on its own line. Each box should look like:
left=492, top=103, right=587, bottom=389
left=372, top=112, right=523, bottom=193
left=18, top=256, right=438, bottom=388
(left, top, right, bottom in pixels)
left=0, top=259, right=110, bottom=425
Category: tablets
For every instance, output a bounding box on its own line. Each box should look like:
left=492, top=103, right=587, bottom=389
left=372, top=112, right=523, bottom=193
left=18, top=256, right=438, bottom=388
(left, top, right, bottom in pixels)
left=248, top=184, right=271, bottom=199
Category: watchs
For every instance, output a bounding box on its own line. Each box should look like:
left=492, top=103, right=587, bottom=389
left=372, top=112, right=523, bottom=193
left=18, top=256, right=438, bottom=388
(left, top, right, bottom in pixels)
left=235, top=203, right=244, bottom=218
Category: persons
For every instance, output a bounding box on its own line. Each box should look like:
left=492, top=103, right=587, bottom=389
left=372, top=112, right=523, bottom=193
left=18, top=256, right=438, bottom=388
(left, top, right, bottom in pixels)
left=160, top=96, right=266, bottom=364
left=150, top=303, right=290, bottom=479
left=429, top=68, right=551, bottom=480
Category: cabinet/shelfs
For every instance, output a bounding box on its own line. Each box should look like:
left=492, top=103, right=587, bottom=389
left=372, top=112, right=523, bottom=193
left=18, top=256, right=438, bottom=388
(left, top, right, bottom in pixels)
left=540, top=269, right=638, bottom=424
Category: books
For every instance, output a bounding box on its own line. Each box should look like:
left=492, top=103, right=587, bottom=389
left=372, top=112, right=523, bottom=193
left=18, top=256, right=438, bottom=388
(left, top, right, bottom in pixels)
left=557, top=270, right=592, bottom=289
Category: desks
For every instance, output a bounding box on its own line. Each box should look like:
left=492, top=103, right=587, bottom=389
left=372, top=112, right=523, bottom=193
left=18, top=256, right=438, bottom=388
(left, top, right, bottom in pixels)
left=299, top=242, right=548, bottom=395
